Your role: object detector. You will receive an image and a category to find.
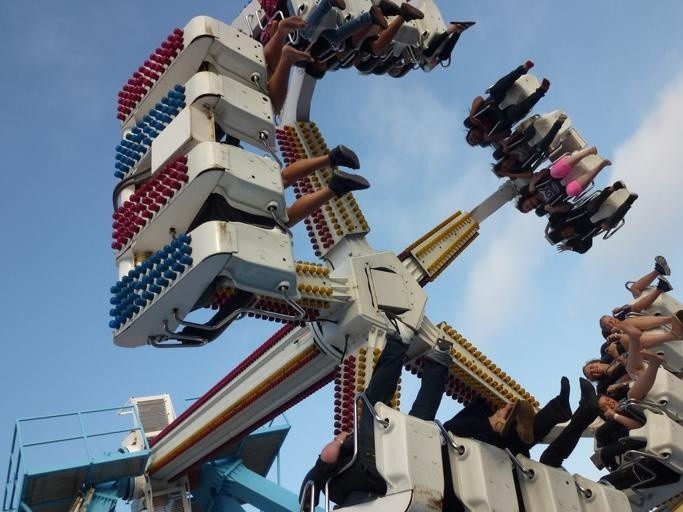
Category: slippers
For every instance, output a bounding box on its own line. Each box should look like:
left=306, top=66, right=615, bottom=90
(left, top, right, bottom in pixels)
left=450, top=21, right=475, bottom=31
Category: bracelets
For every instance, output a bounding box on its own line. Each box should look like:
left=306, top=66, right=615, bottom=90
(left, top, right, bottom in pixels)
left=331, top=436, right=344, bottom=444
left=612, top=413, right=619, bottom=421
left=570, top=245, right=573, bottom=251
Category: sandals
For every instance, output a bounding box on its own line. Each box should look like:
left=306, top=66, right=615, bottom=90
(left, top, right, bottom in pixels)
left=490, top=397, right=521, bottom=438
left=670, top=310, right=683, bottom=339
left=516, top=400, right=535, bottom=443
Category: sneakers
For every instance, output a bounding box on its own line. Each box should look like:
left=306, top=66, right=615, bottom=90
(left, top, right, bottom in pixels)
left=656, top=275, right=673, bottom=293
left=379, top=310, right=420, bottom=341
left=433, top=336, right=455, bottom=355
left=654, top=255, right=671, bottom=276
left=579, top=377, right=599, bottom=416
left=329, top=144, right=361, bottom=169
left=328, top=168, right=371, bottom=199
left=369, top=0, right=424, bottom=30
left=523, top=60, right=534, bottom=70
left=330, top=0, right=347, bottom=10
left=540, top=77, right=550, bottom=94
left=613, top=180, right=626, bottom=192
left=625, top=193, right=638, bottom=205
left=559, top=376, right=572, bottom=419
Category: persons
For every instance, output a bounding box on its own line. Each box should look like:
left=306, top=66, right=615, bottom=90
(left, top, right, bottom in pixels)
left=435, top=398, right=535, bottom=512
left=512, top=145, right=611, bottom=215
left=488, top=113, right=567, bottom=179
left=579, top=309, right=682, bottom=382
left=543, top=180, right=638, bottom=254
left=591, top=321, right=664, bottom=436
left=297, top=310, right=452, bottom=512
left=296, top=0, right=476, bottom=80
left=463, top=58, right=551, bottom=147
left=261, top=15, right=315, bottom=117
left=526, top=376, right=599, bottom=470
left=279, top=143, right=371, bottom=228
left=599, top=256, right=674, bottom=332
